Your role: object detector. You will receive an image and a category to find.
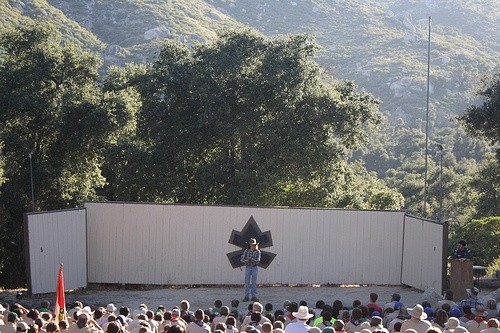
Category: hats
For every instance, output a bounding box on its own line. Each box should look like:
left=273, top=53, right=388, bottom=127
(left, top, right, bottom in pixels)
left=105, top=304, right=118, bottom=311
left=407, top=304, right=428, bottom=320
left=245, top=238, right=259, bottom=245
left=455, top=326, right=468, bottom=333
left=471, top=305, right=489, bottom=318
left=466, top=287, right=479, bottom=297
left=391, top=293, right=400, bottom=298
left=444, top=317, right=459, bottom=328
left=0, top=304, right=6, bottom=311
left=7, top=312, right=19, bottom=321
left=292, top=306, right=314, bottom=319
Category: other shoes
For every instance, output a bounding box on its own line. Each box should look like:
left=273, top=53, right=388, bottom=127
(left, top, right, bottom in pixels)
left=242, top=297, right=249, bottom=302
left=251, top=297, right=259, bottom=302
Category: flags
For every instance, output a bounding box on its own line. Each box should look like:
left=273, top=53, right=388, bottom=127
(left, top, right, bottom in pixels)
left=55, top=263, right=66, bottom=325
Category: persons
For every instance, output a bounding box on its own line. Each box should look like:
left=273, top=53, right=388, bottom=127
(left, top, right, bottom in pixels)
left=240, top=237, right=262, bottom=302
left=451, top=240, right=471, bottom=258
left=0, top=293, right=500, bottom=333
